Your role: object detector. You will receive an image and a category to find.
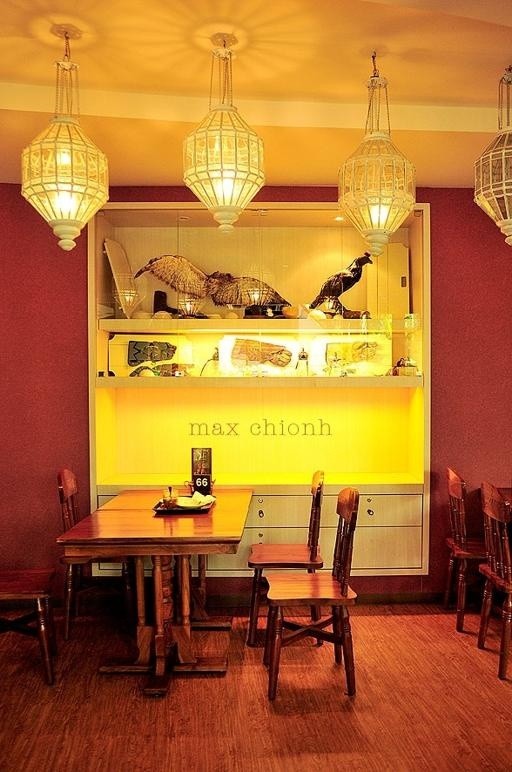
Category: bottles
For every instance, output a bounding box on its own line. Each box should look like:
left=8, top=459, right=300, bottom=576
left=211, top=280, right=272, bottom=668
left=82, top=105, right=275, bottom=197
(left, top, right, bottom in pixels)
left=296, top=347, right=309, bottom=377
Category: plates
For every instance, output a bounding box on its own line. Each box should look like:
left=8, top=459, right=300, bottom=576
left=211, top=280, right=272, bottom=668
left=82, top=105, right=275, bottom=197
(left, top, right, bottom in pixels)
left=178, top=498, right=206, bottom=509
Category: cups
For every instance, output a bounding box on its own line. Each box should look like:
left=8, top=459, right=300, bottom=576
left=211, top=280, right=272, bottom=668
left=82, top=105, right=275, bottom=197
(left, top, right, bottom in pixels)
left=163, top=489, right=179, bottom=509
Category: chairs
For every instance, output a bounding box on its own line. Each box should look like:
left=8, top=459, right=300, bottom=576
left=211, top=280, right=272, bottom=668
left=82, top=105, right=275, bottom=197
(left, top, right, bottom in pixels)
left=477, top=479, right=511, bottom=681
left=56, top=471, right=137, bottom=635
left=264, top=486, right=362, bottom=704
left=245, top=470, right=328, bottom=649
left=441, top=465, right=494, bottom=632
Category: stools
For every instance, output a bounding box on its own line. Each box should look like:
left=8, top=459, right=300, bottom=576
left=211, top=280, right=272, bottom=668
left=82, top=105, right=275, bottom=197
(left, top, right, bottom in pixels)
left=1, top=567, right=63, bottom=686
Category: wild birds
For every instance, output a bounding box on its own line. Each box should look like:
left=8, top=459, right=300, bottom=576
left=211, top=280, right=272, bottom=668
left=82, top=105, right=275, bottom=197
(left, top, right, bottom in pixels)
left=133, top=254, right=291, bottom=316
left=309, top=252, right=372, bottom=310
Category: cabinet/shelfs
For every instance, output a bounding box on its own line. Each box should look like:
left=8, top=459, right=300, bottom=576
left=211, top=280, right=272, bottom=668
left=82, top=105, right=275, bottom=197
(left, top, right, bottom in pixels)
left=87, top=200, right=431, bottom=577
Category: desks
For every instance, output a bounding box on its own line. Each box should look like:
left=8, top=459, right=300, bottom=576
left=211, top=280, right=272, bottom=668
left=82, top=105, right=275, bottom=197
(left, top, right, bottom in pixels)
left=52, top=488, right=257, bottom=697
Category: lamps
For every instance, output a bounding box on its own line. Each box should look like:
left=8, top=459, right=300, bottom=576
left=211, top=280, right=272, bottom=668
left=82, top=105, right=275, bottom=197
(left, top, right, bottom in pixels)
left=20, top=31, right=111, bottom=253
left=335, top=55, right=420, bottom=258
left=470, top=61, right=512, bottom=255
left=179, top=42, right=269, bottom=235
